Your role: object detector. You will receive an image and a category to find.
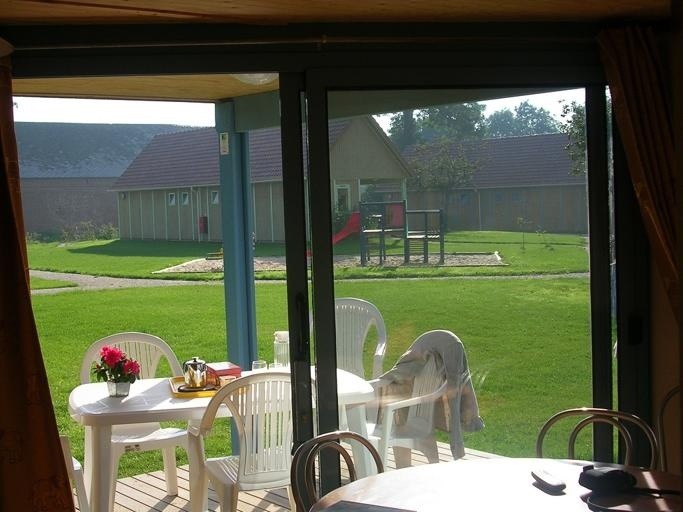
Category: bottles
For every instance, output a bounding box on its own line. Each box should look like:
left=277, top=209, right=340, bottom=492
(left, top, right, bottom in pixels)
left=182, top=356, right=207, bottom=389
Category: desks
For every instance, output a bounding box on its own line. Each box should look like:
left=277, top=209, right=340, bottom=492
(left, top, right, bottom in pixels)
left=311, top=457, right=683, bottom=512
left=67, top=364, right=375, bottom=512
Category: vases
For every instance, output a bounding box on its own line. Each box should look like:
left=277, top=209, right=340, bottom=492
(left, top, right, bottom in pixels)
left=105, top=379, right=130, bottom=397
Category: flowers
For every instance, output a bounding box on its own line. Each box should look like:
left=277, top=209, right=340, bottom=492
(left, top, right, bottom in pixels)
left=92, top=345, right=141, bottom=383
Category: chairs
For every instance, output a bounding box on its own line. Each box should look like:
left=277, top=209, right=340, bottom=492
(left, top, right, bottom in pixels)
left=185, top=374, right=319, bottom=512
left=291, top=431, right=385, bottom=512
left=537, top=408, right=658, bottom=470
left=62, top=435, right=90, bottom=511
left=82, top=332, right=204, bottom=512
left=367, top=331, right=485, bottom=475
left=333, top=299, right=386, bottom=381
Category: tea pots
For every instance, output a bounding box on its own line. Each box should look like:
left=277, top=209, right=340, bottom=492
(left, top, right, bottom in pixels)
left=273, top=330, right=289, bottom=372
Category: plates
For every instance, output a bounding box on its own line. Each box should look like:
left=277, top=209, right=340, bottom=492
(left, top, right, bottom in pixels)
left=177, top=383, right=215, bottom=391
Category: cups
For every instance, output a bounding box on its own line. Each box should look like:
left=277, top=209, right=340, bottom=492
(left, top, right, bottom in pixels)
left=252, top=360, right=266, bottom=372
left=267, top=363, right=282, bottom=370
